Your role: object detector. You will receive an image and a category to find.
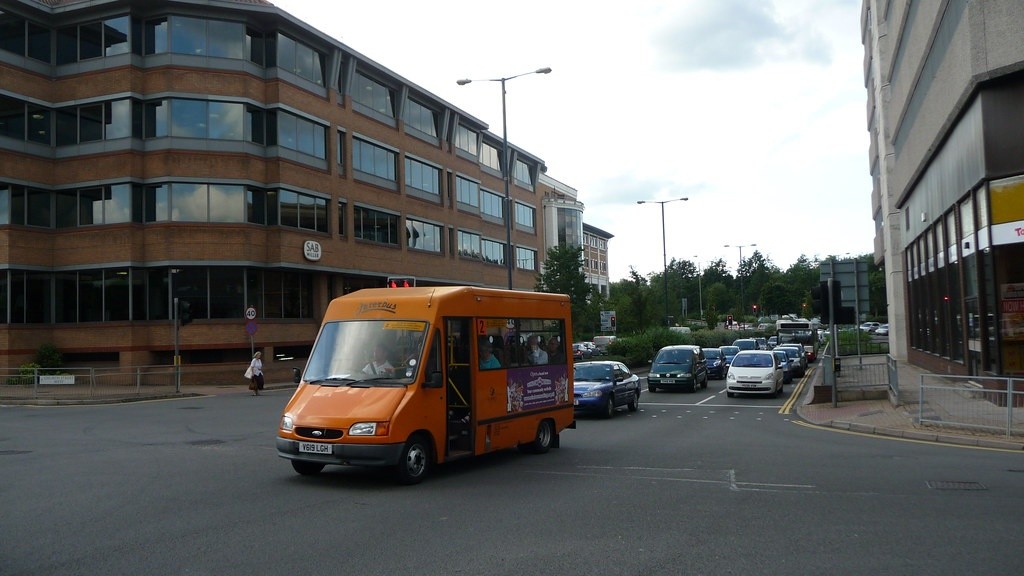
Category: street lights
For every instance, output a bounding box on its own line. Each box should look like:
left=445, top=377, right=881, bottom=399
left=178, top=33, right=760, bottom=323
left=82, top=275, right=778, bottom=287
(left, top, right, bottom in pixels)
left=456, top=68, right=553, bottom=290
left=723, top=243, right=756, bottom=330
left=637, top=197, right=689, bottom=327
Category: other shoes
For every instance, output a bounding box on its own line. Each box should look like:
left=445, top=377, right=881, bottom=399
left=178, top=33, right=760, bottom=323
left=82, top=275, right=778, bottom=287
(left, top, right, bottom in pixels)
left=253, top=391, right=260, bottom=396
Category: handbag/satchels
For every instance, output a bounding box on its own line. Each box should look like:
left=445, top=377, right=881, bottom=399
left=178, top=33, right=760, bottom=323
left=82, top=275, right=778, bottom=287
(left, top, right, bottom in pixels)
left=249, top=378, right=257, bottom=390
left=244, top=365, right=253, bottom=379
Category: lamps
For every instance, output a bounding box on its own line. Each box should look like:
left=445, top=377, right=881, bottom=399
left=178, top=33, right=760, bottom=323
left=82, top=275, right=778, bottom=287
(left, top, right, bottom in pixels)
left=921, top=212, right=926, bottom=222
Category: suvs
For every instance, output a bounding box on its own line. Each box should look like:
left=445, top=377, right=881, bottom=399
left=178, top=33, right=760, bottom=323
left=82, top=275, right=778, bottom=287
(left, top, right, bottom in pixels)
left=731, top=336, right=777, bottom=359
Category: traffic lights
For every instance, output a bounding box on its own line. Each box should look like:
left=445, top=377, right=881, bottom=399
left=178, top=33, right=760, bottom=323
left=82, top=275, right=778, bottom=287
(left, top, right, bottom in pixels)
left=611, top=316, right=616, bottom=327
left=180, top=302, right=192, bottom=326
left=811, top=286, right=827, bottom=319
left=387, top=277, right=414, bottom=288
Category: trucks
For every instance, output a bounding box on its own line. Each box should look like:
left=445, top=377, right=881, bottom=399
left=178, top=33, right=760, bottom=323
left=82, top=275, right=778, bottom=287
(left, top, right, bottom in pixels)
left=777, top=318, right=820, bottom=358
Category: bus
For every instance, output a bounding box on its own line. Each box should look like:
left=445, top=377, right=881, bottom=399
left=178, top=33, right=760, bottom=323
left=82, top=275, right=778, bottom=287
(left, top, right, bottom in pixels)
left=275, top=286, right=574, bottom=484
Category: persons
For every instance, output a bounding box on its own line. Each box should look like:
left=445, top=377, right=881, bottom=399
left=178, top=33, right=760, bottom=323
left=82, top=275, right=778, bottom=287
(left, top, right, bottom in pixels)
left=251, top=352, right=264, bottom=395
left=362, top=344, right=393, bottom=375
left=546, top=338, right=564, bottom=364
left=461, top=341, right=501, bottom=423
left=523, top=336, right=548, bottom=364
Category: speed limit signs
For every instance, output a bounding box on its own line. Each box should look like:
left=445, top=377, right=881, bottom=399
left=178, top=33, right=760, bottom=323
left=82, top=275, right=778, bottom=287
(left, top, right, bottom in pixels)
left=245, top=307, right=256, bottom=320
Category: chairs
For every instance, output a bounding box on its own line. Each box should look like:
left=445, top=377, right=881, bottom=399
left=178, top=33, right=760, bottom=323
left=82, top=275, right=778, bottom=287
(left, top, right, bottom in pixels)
left=453, top=333, right=561, bottom=368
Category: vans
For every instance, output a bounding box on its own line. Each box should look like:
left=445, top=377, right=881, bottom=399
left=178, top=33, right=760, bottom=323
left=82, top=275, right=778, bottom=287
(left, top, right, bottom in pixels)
left=647, top=345, right=708, bottom=393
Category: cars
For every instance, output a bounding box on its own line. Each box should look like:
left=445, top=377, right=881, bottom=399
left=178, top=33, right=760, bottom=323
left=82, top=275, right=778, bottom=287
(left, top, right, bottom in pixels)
left=859, top=322, right=889, bottom=335
left=818, top=328, right=830, bottom=342
left=702, top=346, right=739, bottom=380
left=773, top=343, right=815, bottom=384
left=726, top=350, right=784, bottom=397
left=724, top=313, right=798, bottom=330
left=574, top=360, right=641, bottom=419
left=572, top=336, right=617, bottom=359
